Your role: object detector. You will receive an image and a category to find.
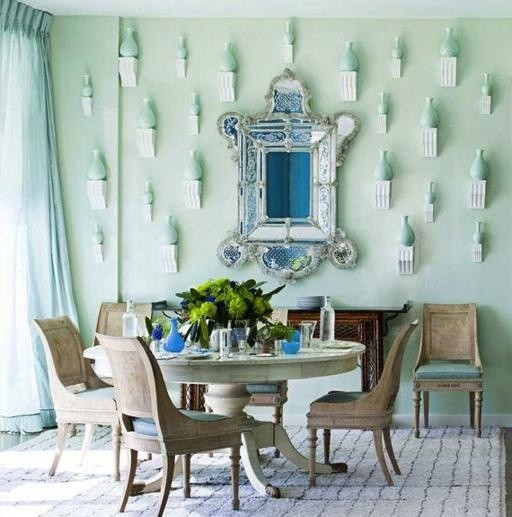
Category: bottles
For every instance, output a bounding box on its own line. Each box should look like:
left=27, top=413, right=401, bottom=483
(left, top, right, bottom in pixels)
left=340, top=26, right=493, bottom=96
left=136, top=93, right=204, bottom=204
left=119, top=27, right=237, bottom=72
left=283, top=21, right=294, bottom=45
left=92, top=222, right=104, bottom=245
left=122, top=299, right=138, bottom=337
left=319, top=295, right=334, bottom=342
left=164, top=318, right=184, bottom=353
left=374, top=91, right=489, bottom=246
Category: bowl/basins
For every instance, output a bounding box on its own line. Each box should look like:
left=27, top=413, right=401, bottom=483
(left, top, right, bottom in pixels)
left=283, top=342, right=300, bottom=354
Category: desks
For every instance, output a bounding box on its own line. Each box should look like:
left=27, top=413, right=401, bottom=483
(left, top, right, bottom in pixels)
left=288, top=298, right=413, bottom=392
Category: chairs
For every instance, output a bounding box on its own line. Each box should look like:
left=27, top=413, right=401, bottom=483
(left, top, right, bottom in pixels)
left=33, top=302, right=152, bottom=481
left=413, top=304, right=483, bottom=438
left=94, top=333, right=257, bottom=517
left=306, top=320, right=419, bottom=486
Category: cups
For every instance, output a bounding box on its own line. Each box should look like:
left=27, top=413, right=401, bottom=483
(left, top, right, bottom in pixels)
left=298, top=320, right=317, bottom=349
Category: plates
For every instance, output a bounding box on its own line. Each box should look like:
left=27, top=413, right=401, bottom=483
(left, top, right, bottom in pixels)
left=295, top=295, right=324, bottom=309
left=323, top=341, right=353, bottom=348
left=183, top=352, right=214, bottom=359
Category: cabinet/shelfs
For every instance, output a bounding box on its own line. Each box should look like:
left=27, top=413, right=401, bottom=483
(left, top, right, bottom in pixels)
left=247, top=378, right=288, bottom=427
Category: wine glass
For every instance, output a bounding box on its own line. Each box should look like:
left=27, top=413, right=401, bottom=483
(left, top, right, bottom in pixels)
left=233, top=328, right=251, bottom=359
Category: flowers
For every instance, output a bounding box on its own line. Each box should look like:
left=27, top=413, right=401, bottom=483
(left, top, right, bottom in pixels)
left=175, top=277, right=286, bottom=349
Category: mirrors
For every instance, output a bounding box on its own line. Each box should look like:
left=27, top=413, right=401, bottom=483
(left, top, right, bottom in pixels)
left=216, top=69, right=358, bottom=281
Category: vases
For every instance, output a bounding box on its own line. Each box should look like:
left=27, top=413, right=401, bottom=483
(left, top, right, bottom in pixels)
left=175, top=37, right=187, bottom=60
left=142, top=182, right=153, bottom=204
left=420, top=98, right=438, bottom=128
left=399, top=216, right=415, bottom=247
left=220, top=41, right=237, bottom=72
left=424, top=183, right=435, bottom=203
left=185, top=150, right=202, bottom=180
left=440, top=27, right=458, bottom=57
left=471, top=147, right=488, bottom=179
left=91, top=222, right=103, bottom=245
left=189, top=94, right=200, bottom=115
left=392, top=36, right=402, bottom=59
left=284, top=21, right=293, bottom=43
left=471, top=222, right=485, bottom=244
left=136, top=98, right=156, bottom=128
left=377, top=93, right=388, bottom=115
left=374, top=149, right=393, bottom=180
left=340, top=41, right=359, bottom=70
left=119, top=28, right=137, bottom=57
left=481, top=73, right=493, bottom=96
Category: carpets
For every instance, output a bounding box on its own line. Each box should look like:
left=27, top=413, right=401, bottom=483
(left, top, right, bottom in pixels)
left=0, top=425, right=506, bottom=517
